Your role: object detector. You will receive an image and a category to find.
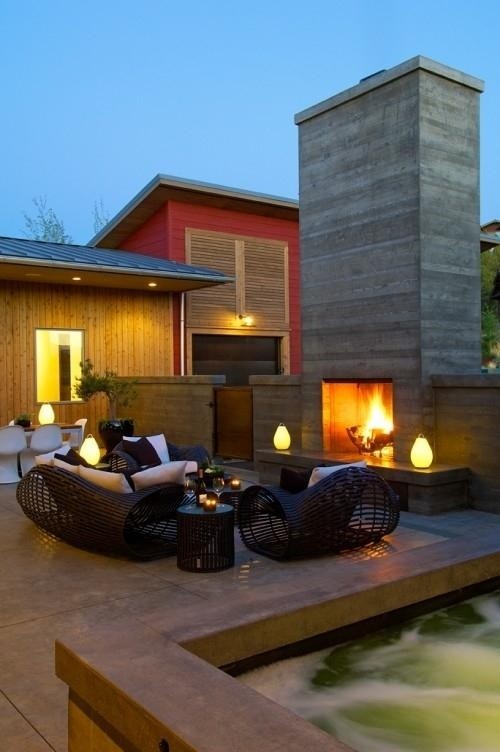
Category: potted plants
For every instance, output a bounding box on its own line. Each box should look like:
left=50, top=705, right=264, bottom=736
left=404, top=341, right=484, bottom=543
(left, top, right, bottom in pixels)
left=73, top=358, right=138, bottom=451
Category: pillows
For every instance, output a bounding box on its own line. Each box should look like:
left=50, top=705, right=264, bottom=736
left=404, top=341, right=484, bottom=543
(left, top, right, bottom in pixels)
left=122, top=432, right=170, bottom=469
left=307, top=461, right=368, bottom=488
left=35, top=448, right=187, bottom=493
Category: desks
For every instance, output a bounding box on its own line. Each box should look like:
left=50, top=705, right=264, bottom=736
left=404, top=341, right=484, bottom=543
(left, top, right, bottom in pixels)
left=195, top=478, right=255, bottom=526
left=175, top=503, right=235, bottom=573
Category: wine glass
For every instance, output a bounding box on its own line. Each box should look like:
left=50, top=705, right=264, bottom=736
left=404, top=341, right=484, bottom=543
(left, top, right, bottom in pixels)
left=183, top=479, right=198, bottom=510
left=213, top=478, right=225, bottom=507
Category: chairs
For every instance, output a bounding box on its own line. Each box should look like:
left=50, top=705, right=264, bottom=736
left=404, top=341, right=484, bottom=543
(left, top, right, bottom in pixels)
left=236, top=466, right=400, bottom=562
left=101, top=434, right=211, bottom=471
left=0, top=418, right=88, bottom=484
left=16, top=464, right=195, bottom=562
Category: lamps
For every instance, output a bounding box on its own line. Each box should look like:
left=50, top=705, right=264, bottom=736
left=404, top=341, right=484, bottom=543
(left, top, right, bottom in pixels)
left=80, top=434, right=100, bottom=465
left=239, top=315, right=255, bottom=326
left=410, top=434, right=434, bottom=468
left=273, top=423, right=291, bottom=450
left=38, top=402, right=55, bottom=425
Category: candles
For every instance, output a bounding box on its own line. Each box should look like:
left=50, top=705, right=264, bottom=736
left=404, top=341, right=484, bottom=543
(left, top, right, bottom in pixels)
left=199, top=493, right=217, bottom=511
left=231, top=479, right=241, bottom=490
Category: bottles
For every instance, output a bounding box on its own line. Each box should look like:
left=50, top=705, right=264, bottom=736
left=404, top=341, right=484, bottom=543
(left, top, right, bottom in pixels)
left=196, top=468, right=208, bottom=508
